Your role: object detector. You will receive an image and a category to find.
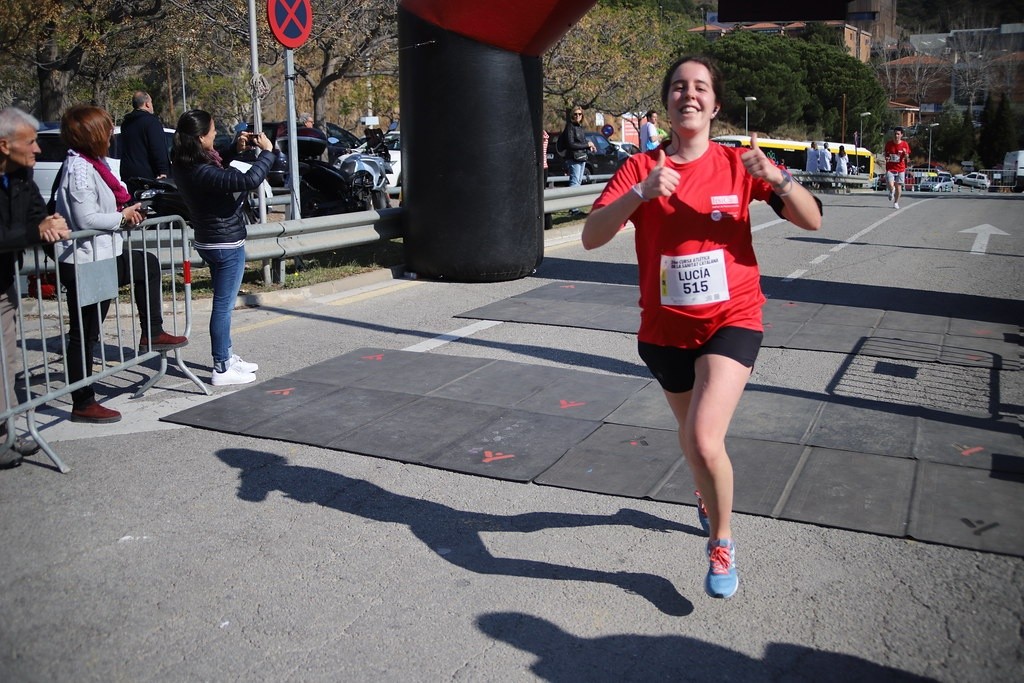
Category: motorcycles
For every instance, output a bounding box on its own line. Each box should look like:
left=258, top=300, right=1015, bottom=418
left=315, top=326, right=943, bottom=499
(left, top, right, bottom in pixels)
left=277, top=122, right=398, bottom=218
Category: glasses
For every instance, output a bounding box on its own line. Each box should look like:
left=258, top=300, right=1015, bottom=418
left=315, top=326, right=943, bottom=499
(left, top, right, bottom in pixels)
left=307, top=120, right=314, bottom=125
left=574, top=112, right=582, bottom=116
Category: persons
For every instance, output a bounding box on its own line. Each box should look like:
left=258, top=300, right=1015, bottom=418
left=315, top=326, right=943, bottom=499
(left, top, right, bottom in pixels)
left=835, top=145, right=849, bottom=188
left=565, top=105, right=589, bottom=219
left=779, top=159, right=787, bottom=169
left=298, top=113, right=314, bottom=128
left=805, top=142, right=832, bottom=188
left=640, top=110, right=663, bottom=152
left=120, top=91, right=170, bottom=192
left=0, top=107, right=72, bottom=470
left=542, top=130, right=553, bottom=230
left=55, top=104, right=189, bottom=423
left=582, top=56, right=824, bottom=599
left=170, top=109, right=276, bottom=386
left=884, top=127, right=911, bottom=208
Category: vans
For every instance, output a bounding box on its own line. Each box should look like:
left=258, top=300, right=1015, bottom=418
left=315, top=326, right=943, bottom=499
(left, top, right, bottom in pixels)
left=1002, top=151, right=1024, bottom=193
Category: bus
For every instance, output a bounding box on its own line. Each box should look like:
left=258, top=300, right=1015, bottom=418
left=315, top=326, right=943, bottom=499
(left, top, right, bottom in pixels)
left=712, top=136, right=874, bottom=183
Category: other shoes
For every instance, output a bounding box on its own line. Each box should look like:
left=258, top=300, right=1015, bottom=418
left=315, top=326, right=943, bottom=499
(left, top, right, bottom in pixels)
left=569, top=209, right=586, bottom=216
left=888, top=187, right=895, bottom=200
left=70, top=402, right=122, bottom=424
left=893, top=202, right=899, bottom=209
left=139, top=331, right=189, bottom=353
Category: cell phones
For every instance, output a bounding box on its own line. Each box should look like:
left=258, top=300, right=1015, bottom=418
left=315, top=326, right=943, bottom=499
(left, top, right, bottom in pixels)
left=246, top=135, right=258, bottom=146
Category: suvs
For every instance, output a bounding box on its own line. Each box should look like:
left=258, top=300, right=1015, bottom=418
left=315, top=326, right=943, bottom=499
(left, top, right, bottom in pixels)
left=229, top=121, right=363, bottom=185
left=955, top=171, right=990, bottom=189
left=34, top=125, right=273, bottom=218
left=546, top=132, right=620, bottom=177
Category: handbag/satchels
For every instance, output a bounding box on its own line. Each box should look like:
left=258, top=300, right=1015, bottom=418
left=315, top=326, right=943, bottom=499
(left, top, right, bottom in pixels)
left=573, top=152, right=588, bottom=162
left=40, top=163, right=67, bottom=260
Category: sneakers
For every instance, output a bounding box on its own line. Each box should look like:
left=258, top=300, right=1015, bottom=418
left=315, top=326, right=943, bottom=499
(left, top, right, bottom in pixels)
left=4, top=433, right=42, bottom=457
left=211, top=364, right=256, bottom=386
left=695, top=490, right=709, bottom=535
left=0, top=443, right=22, bottom=470
left=705, top=538, right=739, bottom=597
left=226, top=353, right=258, bottom=373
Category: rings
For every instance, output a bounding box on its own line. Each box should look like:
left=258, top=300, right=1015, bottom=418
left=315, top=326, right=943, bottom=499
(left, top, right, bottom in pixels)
left=136, top=223, right=139, bottom=227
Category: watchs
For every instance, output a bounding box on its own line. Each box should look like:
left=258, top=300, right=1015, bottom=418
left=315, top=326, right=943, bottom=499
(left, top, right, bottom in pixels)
left=774, top=170, right=791, bottom=190
left=120, top=215, right=127, bottom=228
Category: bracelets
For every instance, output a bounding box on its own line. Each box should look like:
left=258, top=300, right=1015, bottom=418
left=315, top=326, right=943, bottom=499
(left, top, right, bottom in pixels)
left=631, top=183, right=648, bottom=201
left=776, top=181, right=793, bottom=196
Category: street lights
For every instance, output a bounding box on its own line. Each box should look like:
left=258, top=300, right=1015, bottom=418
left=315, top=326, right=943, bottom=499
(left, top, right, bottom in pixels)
left=860, top=112, right=871, bottom=147
left=929, top=123, right=939, bottom=175
left=745, top=96, right=756, bottom=136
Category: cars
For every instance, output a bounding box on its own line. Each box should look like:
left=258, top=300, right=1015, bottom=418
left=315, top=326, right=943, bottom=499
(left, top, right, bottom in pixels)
left=920, top=176, right=955, bottom=193
left=333, top=131, right=401, bottom=195
left=878, top=172, right=916, bottom=192
left=888, top=126, right=922, bottom=136
left=610, top=142, right=642, bottom=157
